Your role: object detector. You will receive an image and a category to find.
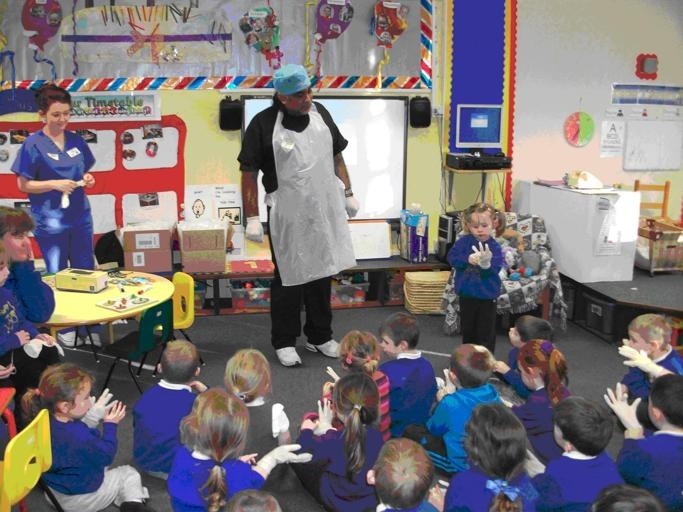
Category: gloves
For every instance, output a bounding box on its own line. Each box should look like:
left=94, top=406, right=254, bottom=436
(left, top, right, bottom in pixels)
left=255, top=443, right=313, bottom=475
left=344, top=194, right=361, bottom=219
left=472, top=242, right=493, bottom=269
left=617, top=345, right=664, bottom=378
left=245, top=214, right=267, bottom=243
left=83, top=388, right=119, bottom=429
left=603, top=382, right=644, bottom=431
left=314, top=398, right=338, bottom=435
left=325, top=365, right=342, bottom=388
left=435, top=368, right=457, bottom=395
left=524, top=449, right=546, bottom=478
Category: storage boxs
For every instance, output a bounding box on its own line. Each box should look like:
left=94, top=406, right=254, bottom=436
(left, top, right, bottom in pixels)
left=584, top=291, right=615, bottom=334
left=230, top=286, right=271, bottom=311
left=330, top=283, right=369, bottom=304
left=115, top=221, right=176, bottom=272
left=178, top=221, right=235, bottom=272
left=193, top=279, right=206, bottom=309
left=386, top=272, right=405, bottom=302
left=561, top=279, right=573, bottom=318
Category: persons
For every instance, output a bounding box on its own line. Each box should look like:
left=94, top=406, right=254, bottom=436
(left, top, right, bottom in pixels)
left=10, top=87, right=109, bottom=349
left=492, top=315, right=555, bottom=387
left=447, top=201, right=507, bottom=353
left=592, top=483, right=665, bottom=512
left=0, top=363, right=16, bottom=379
left=0, top=203, right=56, bottom=324
left=444, top=402, right=537, bottom=511
left=237, top=62, right=359, bottom=368
left=191, top=198, right=207, bottom=220
left=1, top=242, right=61, bottom=404
left=366, top=438, right=440, bottom=512
left=494, top=339, right=576, bottom=466
left=603, top=344, right=683, bottom=512
left=376, top=309, right=438, bottom=437
left=524, top=393, right=625, bottom=512
left=221, top=488, right=284, bottom=512
left=608, top=313, right=683, bottom=429
left=166, top=387, right=313, bottom=512
left=290, top=373, right=385, bottom=512
left=20, top=363, right=157, bottom=512
left=321, top=328, right=391, bottom=442
left=133, top=339, right=209, bottom=480
left=223, top=348, right=292, bottom=481
left=406, top=343, right=507, bottom=476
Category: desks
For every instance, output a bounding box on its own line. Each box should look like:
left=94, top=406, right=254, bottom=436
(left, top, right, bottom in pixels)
left=163, top=251, right=449, bottom=317
left=444, top=166, right=512, bottom=210
left=39, top=268, right=176, bottom=346
left=521, top=179, right=641, bottom=284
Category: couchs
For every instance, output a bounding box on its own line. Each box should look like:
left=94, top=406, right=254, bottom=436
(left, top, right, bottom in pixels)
left=441, top=211, right=568, bottom=337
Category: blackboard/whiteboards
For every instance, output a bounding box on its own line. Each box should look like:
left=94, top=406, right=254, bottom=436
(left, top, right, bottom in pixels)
left=620, top=114, right=681, bottom=176
left=241, top=93, right=408, bottom=221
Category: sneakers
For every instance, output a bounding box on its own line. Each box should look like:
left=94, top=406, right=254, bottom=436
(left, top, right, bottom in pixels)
left=85, top=332, right=101, bottom=348
left=120, top=501, right=155, bottom=512
left=274, top=345, right=304, bottom=369
left=57, top=331, right=84, bottom=348
left=304, top=338, right=342, bottom=358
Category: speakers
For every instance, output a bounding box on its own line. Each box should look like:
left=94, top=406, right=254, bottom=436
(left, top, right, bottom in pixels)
left=218, top=95, right=242, bottom=130
left=409, top=94, right=432, bottom=129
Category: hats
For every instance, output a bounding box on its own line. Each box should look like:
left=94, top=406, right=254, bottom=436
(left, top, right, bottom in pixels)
left=272, top=63, right=311, bottom=94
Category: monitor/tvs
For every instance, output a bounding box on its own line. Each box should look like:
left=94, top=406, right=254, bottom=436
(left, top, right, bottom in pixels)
left=455, top=104, right=504, bottom=156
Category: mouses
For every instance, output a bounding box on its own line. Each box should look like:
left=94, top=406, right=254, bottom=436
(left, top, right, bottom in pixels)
left=495, top=152, right=506, bottom=157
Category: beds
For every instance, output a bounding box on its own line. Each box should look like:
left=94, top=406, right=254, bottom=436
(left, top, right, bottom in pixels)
left=634, top=220, right=683, bottom=276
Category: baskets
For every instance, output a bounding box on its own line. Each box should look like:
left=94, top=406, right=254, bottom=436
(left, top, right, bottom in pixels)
left=403, top=270, right=453, bottom=315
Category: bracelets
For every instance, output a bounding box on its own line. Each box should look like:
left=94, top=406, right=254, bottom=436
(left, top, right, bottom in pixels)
left=344, top=188, right=353, bottom=197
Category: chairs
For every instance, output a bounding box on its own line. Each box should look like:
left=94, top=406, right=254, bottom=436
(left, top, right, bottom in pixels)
left=138, top=271, right=204, bottom=376
left=40, top=272, right=99, bottom=360
left=634, top=180, right=676, bottom=225
left=98, top=299, right=173, bottom=397
left=0, top=406, right=64, bottom=512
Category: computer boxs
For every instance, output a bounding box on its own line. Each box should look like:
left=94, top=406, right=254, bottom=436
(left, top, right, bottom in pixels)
left=437, top=214, right=458, bottom=262
